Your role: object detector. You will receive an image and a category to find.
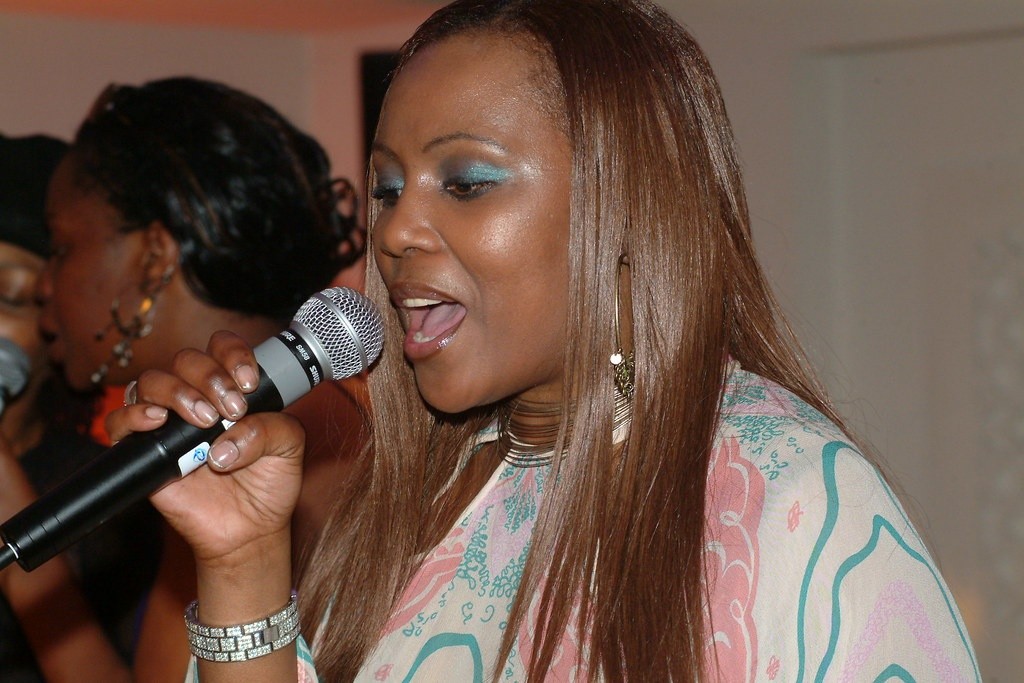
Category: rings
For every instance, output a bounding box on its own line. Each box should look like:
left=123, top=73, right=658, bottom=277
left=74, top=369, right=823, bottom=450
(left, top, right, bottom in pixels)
left=125, top=380, right=141, bottom=404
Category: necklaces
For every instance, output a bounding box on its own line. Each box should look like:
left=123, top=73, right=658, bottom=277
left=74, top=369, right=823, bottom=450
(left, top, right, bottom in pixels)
left=495, top=358, right=649, bottom=471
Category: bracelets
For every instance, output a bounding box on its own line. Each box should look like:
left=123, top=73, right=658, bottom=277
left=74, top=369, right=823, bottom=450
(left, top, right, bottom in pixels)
left=182, top=588, right=303, bottom=667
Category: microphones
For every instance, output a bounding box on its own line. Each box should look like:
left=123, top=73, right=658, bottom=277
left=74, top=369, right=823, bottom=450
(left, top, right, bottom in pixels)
left=0, top=286, right=384, bottom=576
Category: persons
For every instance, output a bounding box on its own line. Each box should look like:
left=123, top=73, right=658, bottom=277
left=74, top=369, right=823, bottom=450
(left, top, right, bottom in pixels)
left=0, top=72, right=374, bottom=683
left=102, top=0, right=981, bottom=683
